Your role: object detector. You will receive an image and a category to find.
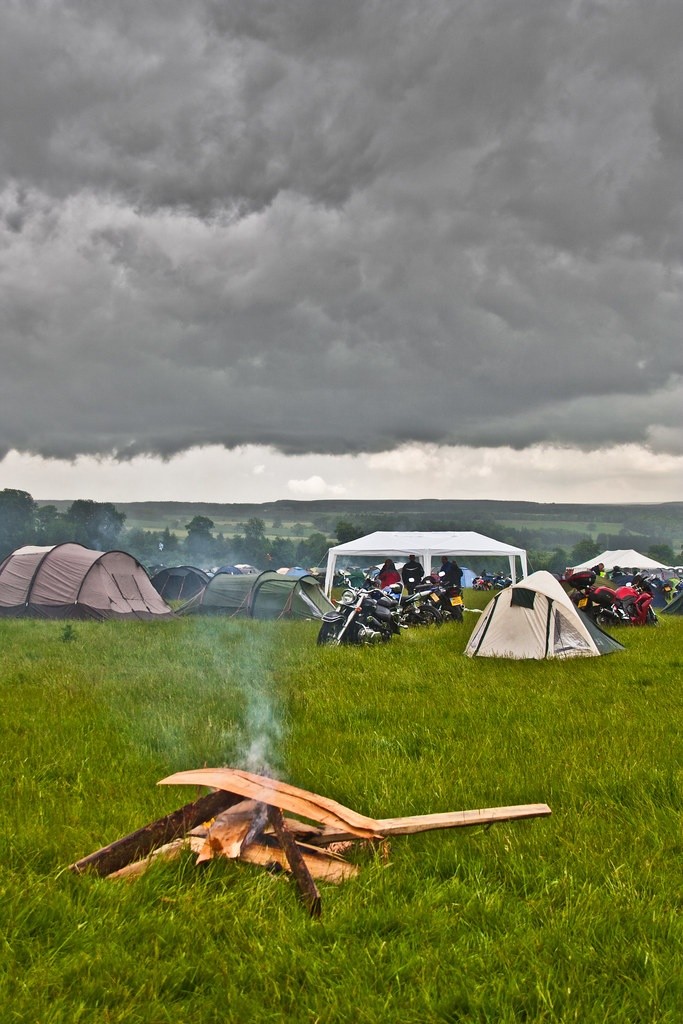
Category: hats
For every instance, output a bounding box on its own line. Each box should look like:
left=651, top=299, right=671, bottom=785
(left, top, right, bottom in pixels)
left=409, top=555, right=415, bottom=559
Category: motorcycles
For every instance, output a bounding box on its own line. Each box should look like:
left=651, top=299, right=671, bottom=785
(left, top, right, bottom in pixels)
left=567, top=568, right=659, bottom=627
left=472, top=571, right=519, bottom=591
left=633, top=568, right=682, bottom=606
left=316, top=567, right=465, bottom=651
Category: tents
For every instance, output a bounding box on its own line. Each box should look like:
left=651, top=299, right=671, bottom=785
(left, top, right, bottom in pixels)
left=0, top=542, right=175, bottom=623
left=151, top=566, right=211, bottom=601
left=324, top=530, right=527, bottom=601
left=176, top=569, right=337, bottom=621
left=464, top=570, right=625, bottom=661
left=567, top=549, right=670, bottom=568
left=143, top=564, right=478, bottom=589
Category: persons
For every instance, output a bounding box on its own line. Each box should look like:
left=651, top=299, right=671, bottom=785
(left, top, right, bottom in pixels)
left=379, top=559, right=401, bottom=590
left=591, top=563, right=604, bottom=576
left=403, top=554, right=424, bottom=594
left=447, top=561, right=463, bottom=588
left=440, top=556, right=451, bottom=580
